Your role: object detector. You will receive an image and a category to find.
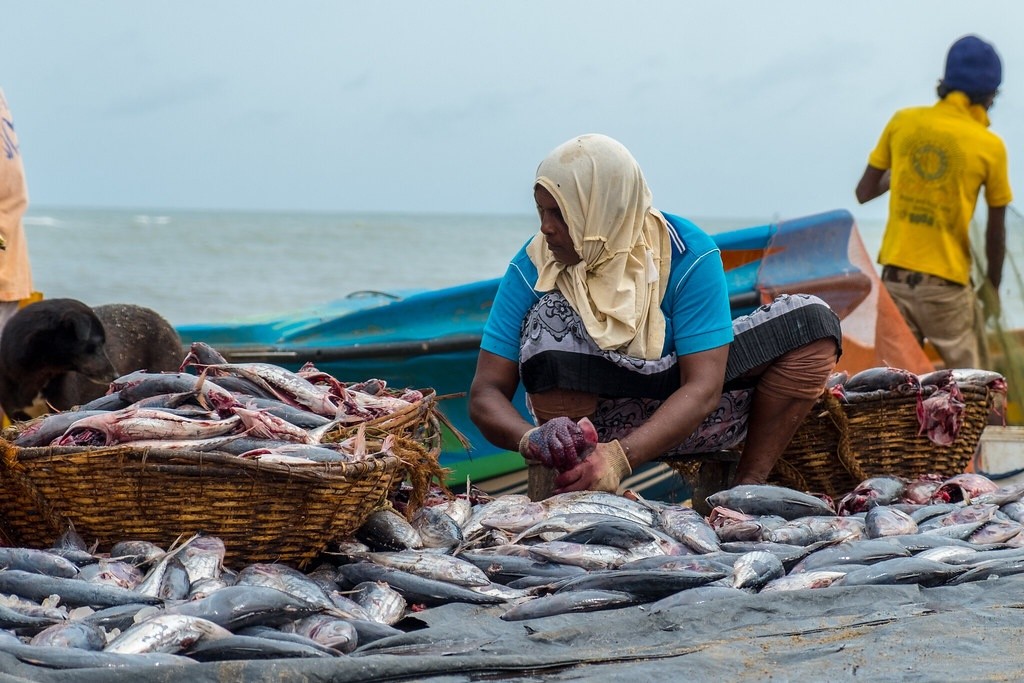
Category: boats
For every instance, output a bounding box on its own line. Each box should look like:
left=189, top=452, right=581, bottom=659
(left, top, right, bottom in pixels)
left=174, top=207, right=938, bottom=495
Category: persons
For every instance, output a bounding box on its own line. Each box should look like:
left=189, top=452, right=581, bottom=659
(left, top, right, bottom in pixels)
left=468, top=134, right=842, bottom=488
left=855, top=37, right=1013, bottom=372
left=0, top=86, right=31, bottom=337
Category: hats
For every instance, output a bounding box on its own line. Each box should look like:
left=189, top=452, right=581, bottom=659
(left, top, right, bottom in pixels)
left=942, top=35, right=1001, bottom=94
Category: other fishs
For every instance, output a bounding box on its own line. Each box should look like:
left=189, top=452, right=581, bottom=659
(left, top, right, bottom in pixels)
left=0, top=338, right=1024, bottom=682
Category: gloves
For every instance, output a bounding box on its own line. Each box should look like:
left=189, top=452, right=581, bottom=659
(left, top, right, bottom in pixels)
left=519, top=416, right=588, bottom=473
left=552, top=440, right=633, bottom=498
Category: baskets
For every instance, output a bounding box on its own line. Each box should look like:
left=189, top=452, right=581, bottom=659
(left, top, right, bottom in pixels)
left=0, top=387, right=436, bottom=574
left=773, top=384, right=995, bottom=508
